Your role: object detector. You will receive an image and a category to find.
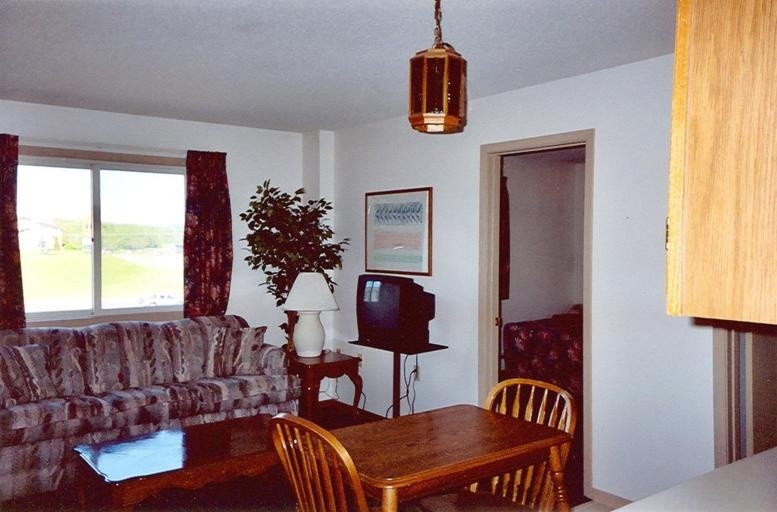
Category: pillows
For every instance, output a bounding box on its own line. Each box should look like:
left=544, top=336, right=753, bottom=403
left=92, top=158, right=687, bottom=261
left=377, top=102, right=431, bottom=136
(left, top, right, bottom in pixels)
left=204, top=325, right=267, bottom=379
left=0, top=343, right=62, bottom=406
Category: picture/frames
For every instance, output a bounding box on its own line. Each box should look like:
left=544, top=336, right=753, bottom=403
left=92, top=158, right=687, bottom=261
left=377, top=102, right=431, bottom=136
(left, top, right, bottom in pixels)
left=364, top=186, right=433, bottom=276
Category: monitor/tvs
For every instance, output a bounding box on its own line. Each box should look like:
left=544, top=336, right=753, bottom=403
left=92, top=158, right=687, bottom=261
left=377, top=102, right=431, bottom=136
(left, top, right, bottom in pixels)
left=356, top=274, right=435, bottom=350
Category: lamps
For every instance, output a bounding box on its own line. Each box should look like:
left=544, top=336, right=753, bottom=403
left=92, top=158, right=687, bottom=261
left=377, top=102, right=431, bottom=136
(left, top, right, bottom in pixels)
left=407, top=0, right=468, bottom=135
left=281, top=272, right=341, bottom=358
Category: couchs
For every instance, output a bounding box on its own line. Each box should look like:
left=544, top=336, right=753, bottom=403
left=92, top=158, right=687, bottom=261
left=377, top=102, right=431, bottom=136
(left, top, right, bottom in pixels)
left=0, top=314, right=302, bottom=512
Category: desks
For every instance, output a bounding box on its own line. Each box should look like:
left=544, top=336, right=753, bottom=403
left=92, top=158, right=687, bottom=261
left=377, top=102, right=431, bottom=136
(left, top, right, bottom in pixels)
left=348, top=341, right=448, bottom=419
left=280, top=343, right=365, bottom=425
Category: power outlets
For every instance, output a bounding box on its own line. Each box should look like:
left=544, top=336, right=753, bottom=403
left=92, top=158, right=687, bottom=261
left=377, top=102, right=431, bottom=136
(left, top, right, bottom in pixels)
left=413, top=365, right=422, bottom=382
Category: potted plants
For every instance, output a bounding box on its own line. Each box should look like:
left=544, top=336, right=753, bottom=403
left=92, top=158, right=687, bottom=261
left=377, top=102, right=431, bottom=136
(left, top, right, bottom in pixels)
left=237, top=178, right=352, bottom=369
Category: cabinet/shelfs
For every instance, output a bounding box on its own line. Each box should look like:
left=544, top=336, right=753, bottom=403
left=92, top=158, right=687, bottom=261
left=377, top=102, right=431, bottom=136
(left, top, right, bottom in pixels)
left=665, top=0, right=777, bottom=325
